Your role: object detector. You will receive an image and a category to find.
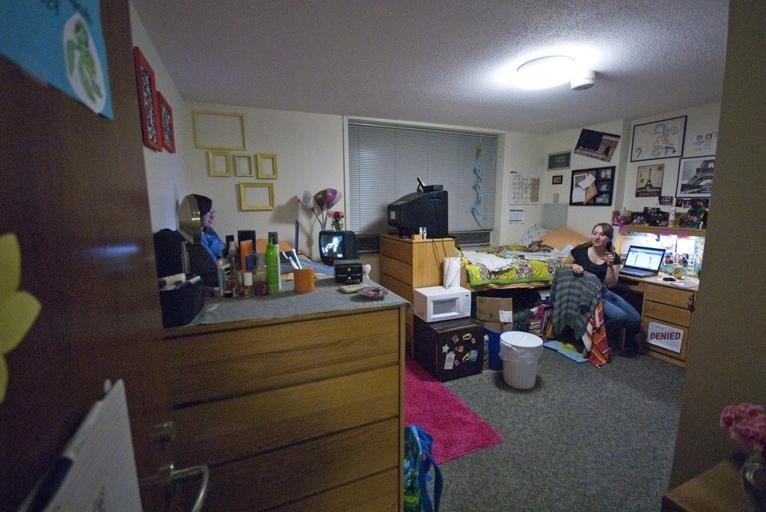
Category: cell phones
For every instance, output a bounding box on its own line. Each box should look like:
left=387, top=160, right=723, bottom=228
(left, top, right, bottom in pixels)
left=663, top=277, right=675, bottom=281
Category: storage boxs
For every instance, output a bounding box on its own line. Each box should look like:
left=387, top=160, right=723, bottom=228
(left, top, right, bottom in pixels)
left=484, top=321, right=513, bottom=333
left=474, top=294, right=514, bottom=323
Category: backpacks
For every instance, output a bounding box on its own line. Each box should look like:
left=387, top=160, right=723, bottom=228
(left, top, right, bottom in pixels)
left=404, top=423, right=443, bottom=511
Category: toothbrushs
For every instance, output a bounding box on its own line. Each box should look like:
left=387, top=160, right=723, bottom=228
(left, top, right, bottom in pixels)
left=280, top=250, right=297, bottom=269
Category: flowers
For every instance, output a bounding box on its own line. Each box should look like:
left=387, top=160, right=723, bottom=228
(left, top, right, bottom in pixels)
left=325, top=210, right=345, bottom=219
left=720, top=402, right=766, bottom=454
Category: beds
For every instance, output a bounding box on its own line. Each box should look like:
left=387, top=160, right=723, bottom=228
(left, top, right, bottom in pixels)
left=460, top=225, right=594, bottom=290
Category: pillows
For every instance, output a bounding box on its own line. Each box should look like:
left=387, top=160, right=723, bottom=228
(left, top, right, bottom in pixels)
left=541, top=227, right=592, bottom=253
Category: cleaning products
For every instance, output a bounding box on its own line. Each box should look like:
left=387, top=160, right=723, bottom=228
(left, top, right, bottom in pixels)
left=244, top=271, right=254, bottom=299
left=266, top=237, right=278, bottom=294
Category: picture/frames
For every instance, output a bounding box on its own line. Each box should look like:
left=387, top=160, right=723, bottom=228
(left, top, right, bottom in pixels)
left=635, top=163, right=665, bottom=198
left=134, top=47, right=163, bottom=151
left=630, top=212, right=647, bottom=226
left=547, top=150, right=572, bottom=170
left=157, top=92, right=175, bottom=153
left=194, top=110, right=278, bottom=211
left=552, top=175, right=563, bottom=185
left=569, top=166, right=616, bottom=206
left=630, top=115, right=687, bottom=162
left=675, top=154, right=716, bottom=198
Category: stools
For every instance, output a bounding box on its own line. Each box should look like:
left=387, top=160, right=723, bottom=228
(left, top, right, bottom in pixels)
left=414, top=317, right=486, bottom=383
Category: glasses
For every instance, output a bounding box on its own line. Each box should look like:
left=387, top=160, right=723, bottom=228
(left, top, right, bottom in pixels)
left=206, top=210, right=216, bottom=215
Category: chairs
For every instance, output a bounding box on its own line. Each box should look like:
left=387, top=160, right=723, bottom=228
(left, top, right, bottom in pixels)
left=549, top=266, right=633, bottom=358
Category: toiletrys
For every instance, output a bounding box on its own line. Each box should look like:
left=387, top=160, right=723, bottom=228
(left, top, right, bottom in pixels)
left=241, top=270, right=253, bottom=297
left=221, top=262, right=233, bottom=299
left=254, top=264, right=268, bottom=297
left=216, top=259, right=224, bottom=296
left=267, top=236, right=281, bottom=292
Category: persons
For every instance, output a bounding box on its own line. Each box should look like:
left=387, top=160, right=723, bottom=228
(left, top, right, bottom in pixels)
left=564, top=223, right=641, bottom=359
left=327, top=237, right=344, bottom=257
left=177, top=194, right=372, bottom=276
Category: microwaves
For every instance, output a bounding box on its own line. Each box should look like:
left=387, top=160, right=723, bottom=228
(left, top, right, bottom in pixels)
left=413, top=286, right=472, bottom=323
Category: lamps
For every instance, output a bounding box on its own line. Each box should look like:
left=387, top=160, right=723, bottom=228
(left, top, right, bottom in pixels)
left=299, top=188, right=343, bottom=229
left=516, top=55, right=597, bottom=91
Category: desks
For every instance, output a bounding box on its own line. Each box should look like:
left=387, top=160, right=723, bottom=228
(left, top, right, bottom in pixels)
left=662, top=453, right=766, bottom=512
left=604, top=224, right=707, bottom=368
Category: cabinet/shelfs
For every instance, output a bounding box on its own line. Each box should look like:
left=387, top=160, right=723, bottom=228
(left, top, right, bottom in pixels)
left=160, top=303, right=405, bottom=512
left=380, top=233, right=456, bottom=361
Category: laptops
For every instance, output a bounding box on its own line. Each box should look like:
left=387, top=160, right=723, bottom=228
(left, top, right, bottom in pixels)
left=619, top=245, right=666, bottom=278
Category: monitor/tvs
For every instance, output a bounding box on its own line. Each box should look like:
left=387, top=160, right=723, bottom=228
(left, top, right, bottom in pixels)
left=386, top=190, right=448, bottom=238
left=318, top=230, right=358, bottom=266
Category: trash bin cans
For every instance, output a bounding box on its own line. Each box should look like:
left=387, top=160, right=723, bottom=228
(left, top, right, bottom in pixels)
left=500, top=331, right=544, bottom=390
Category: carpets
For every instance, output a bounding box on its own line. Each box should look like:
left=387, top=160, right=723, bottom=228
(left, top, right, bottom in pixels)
left=404, top=353, right=507, bottom=469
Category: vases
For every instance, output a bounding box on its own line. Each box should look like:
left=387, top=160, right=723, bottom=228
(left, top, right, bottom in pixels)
left=740, top=454, right=766, bottom=512
left=331, top=219, right=343, bottom=232
